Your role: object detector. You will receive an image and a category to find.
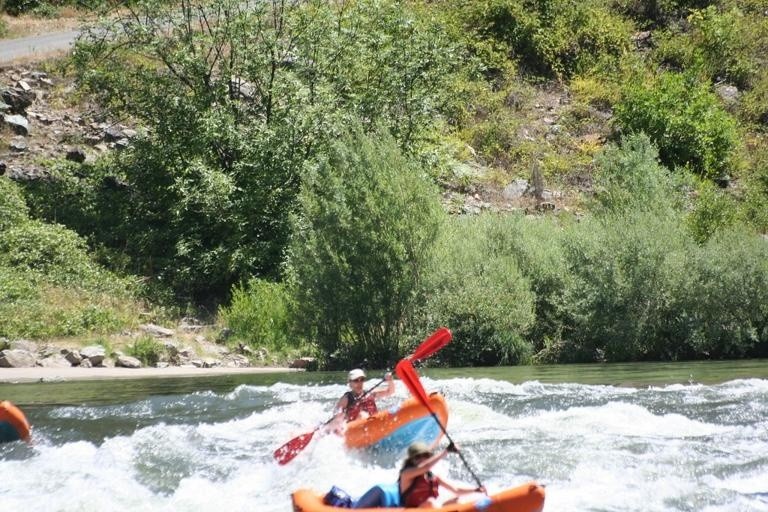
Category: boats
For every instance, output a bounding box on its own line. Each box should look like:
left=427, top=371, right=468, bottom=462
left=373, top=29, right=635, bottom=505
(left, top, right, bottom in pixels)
left=0, top=399, right=31, bottom=443
left=290, top=389, right=545, bottom=512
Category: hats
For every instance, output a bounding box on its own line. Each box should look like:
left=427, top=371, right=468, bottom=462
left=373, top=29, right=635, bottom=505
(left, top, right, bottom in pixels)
left=407, top=441, right=432, bottom=458
left=348, top=368, right=365, bottom=381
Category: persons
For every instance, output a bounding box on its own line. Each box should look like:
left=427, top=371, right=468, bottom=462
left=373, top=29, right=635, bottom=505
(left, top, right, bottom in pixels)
left=399, top=442, right=486, bottom=507
left=333, top=368, right=395, bottom=424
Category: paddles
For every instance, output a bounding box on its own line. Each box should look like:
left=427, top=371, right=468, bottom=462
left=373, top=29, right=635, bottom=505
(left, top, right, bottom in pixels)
left=396, top=359, right=487, bottom=495
left=272, top=327, right=452, bottom=465
